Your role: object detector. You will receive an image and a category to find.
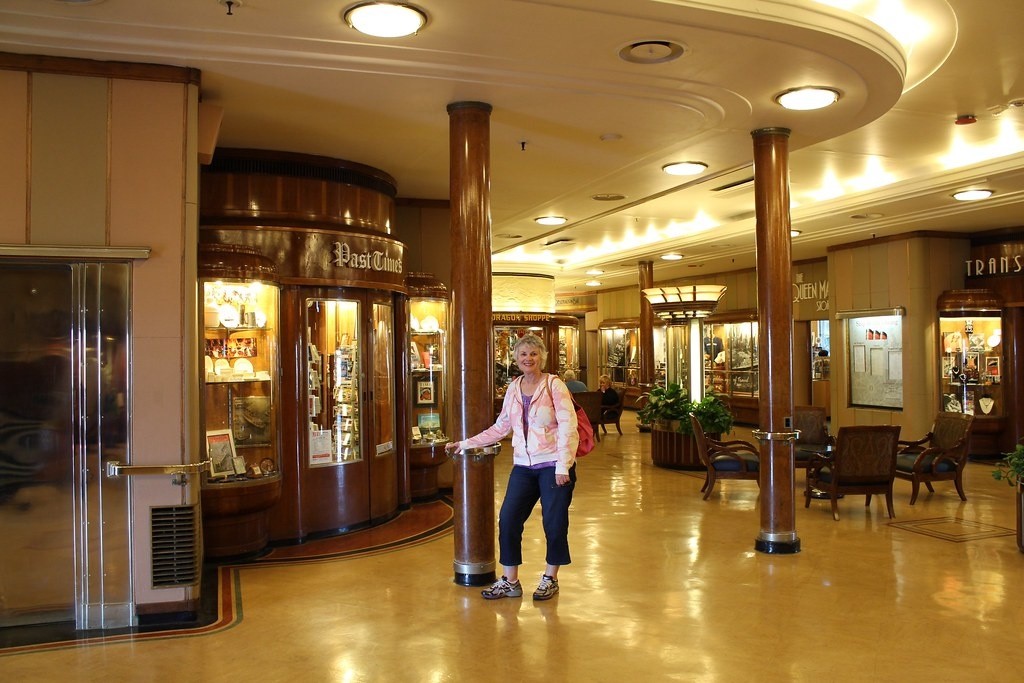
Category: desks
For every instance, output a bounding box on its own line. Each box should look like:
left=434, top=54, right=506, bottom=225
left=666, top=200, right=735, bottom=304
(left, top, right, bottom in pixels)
left=947, top=381, right=989, bottom=413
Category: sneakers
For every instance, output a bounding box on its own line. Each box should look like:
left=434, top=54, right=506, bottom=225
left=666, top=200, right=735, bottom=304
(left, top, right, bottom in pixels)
left=532, top=574, right=559, bottom=600
left=482, top=575, right=522, bottom=599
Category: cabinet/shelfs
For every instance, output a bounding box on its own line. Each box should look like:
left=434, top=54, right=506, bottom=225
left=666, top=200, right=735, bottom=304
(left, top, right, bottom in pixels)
left=202, top=325, right=273, bottom=458
left=410, top=330, right=447, bottom=448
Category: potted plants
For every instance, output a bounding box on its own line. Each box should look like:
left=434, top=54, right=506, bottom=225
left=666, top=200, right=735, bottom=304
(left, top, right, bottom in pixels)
left=636, top=379, right=734, bottom=476
left=991, top=443, right=1024, bottom=556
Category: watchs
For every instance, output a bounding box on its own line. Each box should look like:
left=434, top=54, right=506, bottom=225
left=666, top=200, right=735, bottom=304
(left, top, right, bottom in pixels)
left=602, top=390, right=605, bottom=393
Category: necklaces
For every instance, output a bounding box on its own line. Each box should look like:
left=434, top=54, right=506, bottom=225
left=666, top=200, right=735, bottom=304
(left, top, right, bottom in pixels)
left=982, top=398, right=992, bottom=408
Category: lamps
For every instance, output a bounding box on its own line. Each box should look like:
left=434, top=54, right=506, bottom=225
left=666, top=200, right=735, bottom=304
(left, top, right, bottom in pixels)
left=203, top=280, right=269, bottom=330
left=640, top=284, right=728, bottom=319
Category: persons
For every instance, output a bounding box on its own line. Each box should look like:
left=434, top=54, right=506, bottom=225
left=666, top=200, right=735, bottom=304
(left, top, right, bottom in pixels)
left=818, top=350, right=828, bottom=357
left=563, top=369, right=618, bottom=419
left=703, top=327, right=724, bottom=361
left=445, top=335, right=579, bottom=600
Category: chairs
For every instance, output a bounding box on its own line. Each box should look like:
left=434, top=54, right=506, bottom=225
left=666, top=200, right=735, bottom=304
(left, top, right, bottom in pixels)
left=687, top=411, right=761, bottom=504
left=803, top=423, right=901, bottom=521
left=897, top=409, right=978, bottom=505
left=594, top=388, right=628, bottom=437
left=794, top=404, right=837, bottom=468
left=573, top=390, right=604, bottom=443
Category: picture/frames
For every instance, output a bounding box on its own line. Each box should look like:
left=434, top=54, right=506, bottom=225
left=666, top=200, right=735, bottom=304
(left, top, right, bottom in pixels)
left=413, top=375, right=439, bottom=408
left=206, top=429, right=240, bottom=479
left=942, top=330, right=1000, bottom=378
left=232, top=395, right=272, bottom=448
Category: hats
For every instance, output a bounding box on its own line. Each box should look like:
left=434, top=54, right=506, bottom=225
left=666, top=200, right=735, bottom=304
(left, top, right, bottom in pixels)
left=818, top=350, right=828, bottom=356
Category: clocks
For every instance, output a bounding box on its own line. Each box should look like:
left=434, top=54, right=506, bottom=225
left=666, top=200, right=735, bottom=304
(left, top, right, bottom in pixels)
left=259, top=458, right=275, bottom=472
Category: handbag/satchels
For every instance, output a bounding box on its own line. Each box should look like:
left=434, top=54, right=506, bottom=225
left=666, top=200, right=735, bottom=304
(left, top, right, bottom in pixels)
left=545, top=373, right=595, bottom=457
